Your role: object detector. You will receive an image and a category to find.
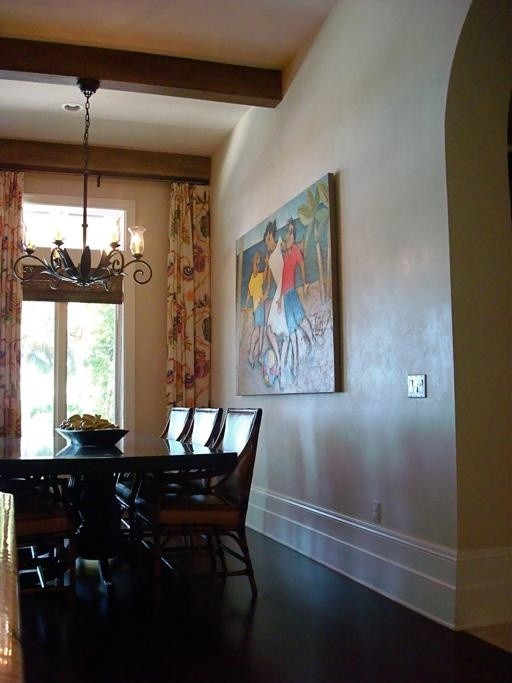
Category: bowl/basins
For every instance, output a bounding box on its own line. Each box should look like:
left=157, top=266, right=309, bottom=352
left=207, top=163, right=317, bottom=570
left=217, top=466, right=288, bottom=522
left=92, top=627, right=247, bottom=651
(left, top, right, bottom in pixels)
left=54, top=426, right=130, bottom=449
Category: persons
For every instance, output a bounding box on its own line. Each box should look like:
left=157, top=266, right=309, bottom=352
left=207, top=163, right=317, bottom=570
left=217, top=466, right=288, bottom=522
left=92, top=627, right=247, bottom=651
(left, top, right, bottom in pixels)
left=243, top=217, right=317, bottom=390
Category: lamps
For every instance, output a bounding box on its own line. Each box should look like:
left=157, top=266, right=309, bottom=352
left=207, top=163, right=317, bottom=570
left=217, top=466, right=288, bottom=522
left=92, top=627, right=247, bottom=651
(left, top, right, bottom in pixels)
left=12, top=75, right=153, bottom=290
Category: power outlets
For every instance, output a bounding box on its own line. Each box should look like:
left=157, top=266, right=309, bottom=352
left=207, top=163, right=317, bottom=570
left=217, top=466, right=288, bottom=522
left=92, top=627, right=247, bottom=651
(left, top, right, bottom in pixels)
left=369, top=500, right=380, bottom=523
